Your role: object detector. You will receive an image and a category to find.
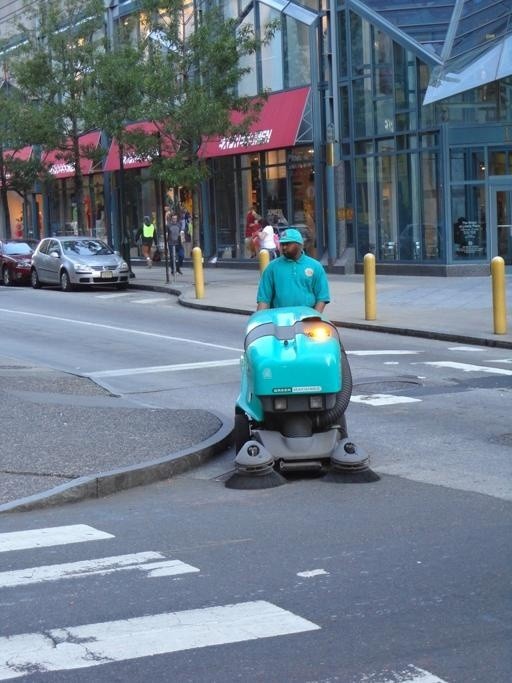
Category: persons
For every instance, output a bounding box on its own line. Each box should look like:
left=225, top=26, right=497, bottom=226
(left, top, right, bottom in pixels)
left=245, top=206, right=258, bottom=259
left=15, top=221, right=24, bottom=239
left=164, top=214, right=185, bottom=277
left=134, top=216, right=158, bottom=269
left=256, top=218, right=276, bottom=261
left=256, top=229, right=331, bottom=315
left=70, top=201, right=79, bottom=236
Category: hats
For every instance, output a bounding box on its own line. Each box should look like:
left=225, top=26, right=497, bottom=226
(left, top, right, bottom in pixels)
left=277, top=227, right=306, bottom=245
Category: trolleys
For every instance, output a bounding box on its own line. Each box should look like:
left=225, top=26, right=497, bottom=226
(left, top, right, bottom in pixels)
left=233, top=306, right=361, bottom=470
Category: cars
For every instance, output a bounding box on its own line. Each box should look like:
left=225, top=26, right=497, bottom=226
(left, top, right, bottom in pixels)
left=399, top=223, right=444, bottom=261
left=0, top=236, right=129, bottom=293
